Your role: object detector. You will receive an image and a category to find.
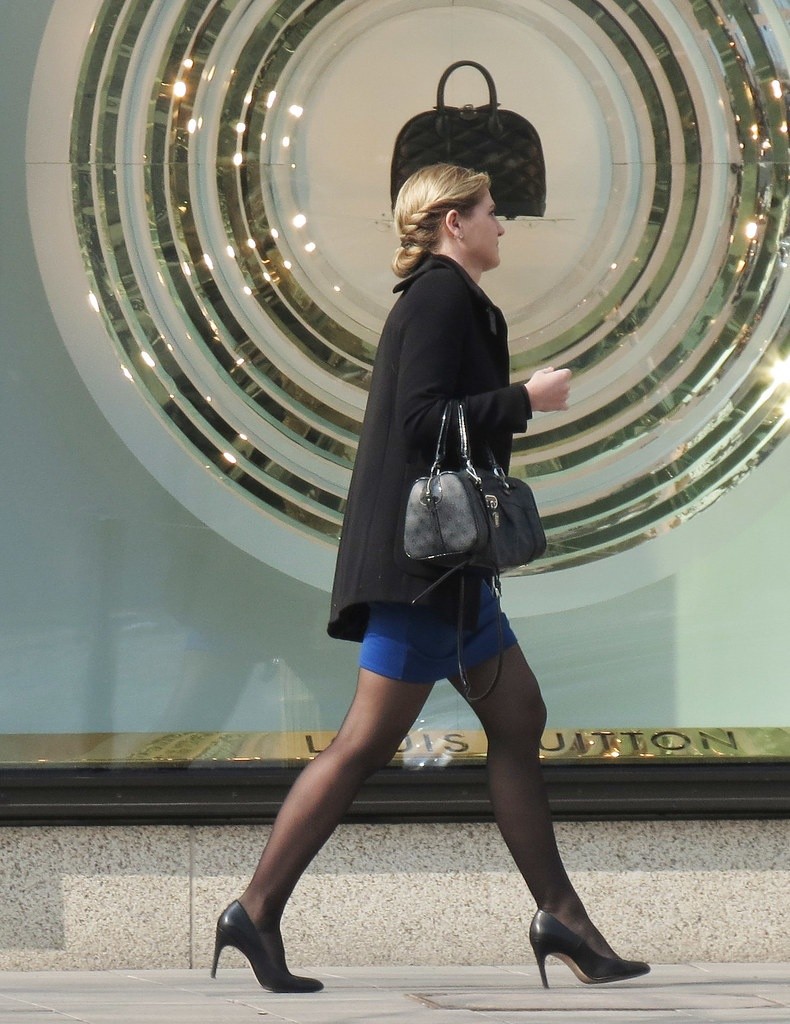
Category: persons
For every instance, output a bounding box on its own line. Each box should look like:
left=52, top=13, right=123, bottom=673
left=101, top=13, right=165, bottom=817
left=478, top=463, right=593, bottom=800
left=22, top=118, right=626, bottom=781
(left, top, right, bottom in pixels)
left=210, top=164, right=652, bottom=994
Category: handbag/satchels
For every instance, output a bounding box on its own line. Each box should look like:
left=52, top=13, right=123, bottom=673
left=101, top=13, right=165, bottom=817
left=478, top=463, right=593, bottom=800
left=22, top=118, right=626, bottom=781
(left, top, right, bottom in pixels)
left=404, top=400, right=547, bottom=576
left=393, top=63, right=548, bottom=219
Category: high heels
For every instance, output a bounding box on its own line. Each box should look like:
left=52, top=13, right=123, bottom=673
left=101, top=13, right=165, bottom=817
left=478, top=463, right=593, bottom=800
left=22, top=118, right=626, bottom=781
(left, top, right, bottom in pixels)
left=211, top=900, right=324, bottom=992
left=530, top=908, right=652, bottom=988
